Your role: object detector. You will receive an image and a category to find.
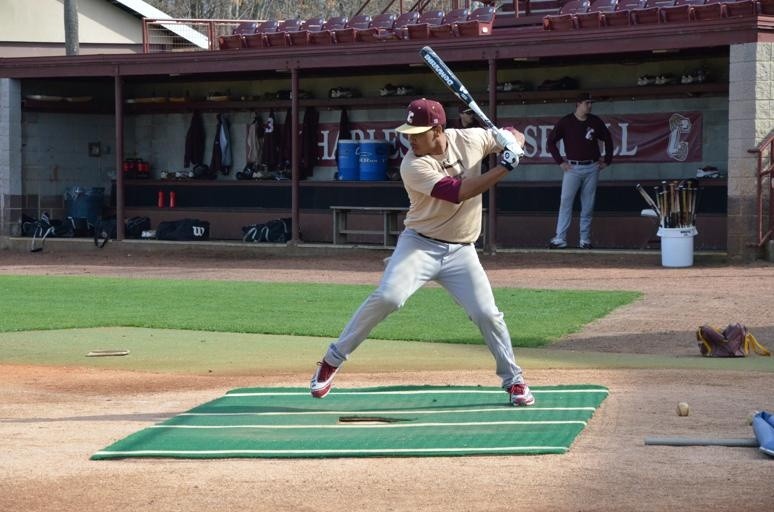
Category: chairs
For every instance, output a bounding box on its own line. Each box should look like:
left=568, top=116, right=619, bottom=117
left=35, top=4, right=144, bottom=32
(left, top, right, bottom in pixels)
left=540, top=2, right=761, bottom=30
left=216, top=1, right=497, bottom=52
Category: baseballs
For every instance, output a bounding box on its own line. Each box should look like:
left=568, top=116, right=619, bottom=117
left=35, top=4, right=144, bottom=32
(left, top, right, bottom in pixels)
left=676, top=402, right=691, bottom=417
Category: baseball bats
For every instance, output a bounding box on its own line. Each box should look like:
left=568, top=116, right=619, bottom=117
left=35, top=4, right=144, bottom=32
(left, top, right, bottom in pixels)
left=654, top=178, right=699, bottom=229
left=635, top=183, right=662, bottom=221
left=420, top=43, right=526, bottom=160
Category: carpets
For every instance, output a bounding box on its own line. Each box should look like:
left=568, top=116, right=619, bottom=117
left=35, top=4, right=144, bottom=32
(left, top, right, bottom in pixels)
left=87, top=384, right=610, bottom=461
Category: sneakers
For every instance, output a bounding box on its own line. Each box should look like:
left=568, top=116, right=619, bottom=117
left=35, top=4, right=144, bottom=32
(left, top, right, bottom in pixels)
left=636, top=69, right=707, bottom=86
left=329, top=86, right=354, bottom=98
left=236, top=166, right=290, bottom=182
left=311, top=357, right=343, bottom=399
left=141, top=229, right=157, bottom=240
left=506, top=381, right=535, bottom=406
left=544, top=239, right=567, bottom=249
left=378, top=83, right=414, bottom=96
left=696, top=166, right=727, bottom=179
left=206, top=88, right=233, bottom=101
left=537, top=78, right=576, bottom=91
left=576, top=240, right=593, bottom=249
left=160, top=164, right=218, bottom=181
left=240, top=90, right=313, bottom=101
left=486, top=80, right=527, bottom=93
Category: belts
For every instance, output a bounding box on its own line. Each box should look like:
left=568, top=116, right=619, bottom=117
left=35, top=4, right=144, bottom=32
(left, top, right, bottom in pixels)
left=569, top=158, right=599, bottom=166
left=418, top=231, right=472, bottom=245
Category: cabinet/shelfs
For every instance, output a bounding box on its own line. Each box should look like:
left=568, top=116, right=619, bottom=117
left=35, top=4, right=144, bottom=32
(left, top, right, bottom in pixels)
left=20, top=81, right=729, bottom=251
left=2, top=193, right=70, bottom=237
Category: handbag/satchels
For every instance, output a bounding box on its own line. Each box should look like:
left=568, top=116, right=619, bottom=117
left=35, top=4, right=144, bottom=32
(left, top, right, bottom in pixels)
left=22, top=213, right=151, bottom=239
left=241, top=217, right=292, bottom=244
left=156, top=218, right=210, bottom=241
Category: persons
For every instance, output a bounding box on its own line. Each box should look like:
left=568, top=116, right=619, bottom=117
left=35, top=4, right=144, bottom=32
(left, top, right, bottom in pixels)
left=309, top=98, right=541, bottom=410
left=548, top=92, right=615, bottom=249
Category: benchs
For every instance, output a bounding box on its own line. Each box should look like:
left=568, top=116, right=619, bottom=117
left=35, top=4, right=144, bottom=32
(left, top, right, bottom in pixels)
left=330, top=206, right=489, bottom=251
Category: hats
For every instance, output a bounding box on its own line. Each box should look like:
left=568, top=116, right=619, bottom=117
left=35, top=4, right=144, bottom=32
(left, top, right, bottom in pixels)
left=575, top=92, right=596, bottom=107
left=458, top=104, right=474, bottom=114
left=394, top=98, right=447, bottom=135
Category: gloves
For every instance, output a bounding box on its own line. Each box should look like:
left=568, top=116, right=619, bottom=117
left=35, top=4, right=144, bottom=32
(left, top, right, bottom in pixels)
left=497, top=150, right=520, bottom=172
left=492, top=129, right=516, bottom=150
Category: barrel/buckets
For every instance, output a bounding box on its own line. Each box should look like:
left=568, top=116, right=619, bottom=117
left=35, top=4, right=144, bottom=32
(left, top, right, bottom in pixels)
left=338, top=139, right=360, bottom=182
left=656, top=224, right=698, bottom=266
left=356, top=138, right=391, bottom=181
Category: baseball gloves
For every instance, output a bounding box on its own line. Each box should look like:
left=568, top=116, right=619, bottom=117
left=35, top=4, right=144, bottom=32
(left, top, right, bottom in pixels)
left=696, top=324, right=748, bottom=358
